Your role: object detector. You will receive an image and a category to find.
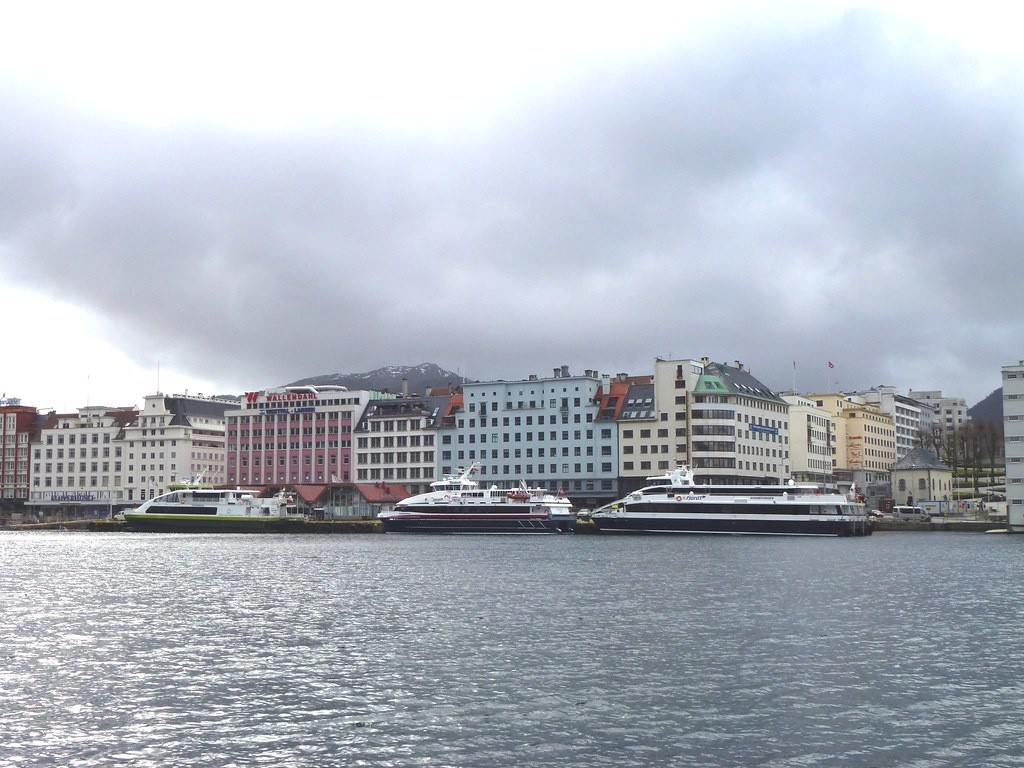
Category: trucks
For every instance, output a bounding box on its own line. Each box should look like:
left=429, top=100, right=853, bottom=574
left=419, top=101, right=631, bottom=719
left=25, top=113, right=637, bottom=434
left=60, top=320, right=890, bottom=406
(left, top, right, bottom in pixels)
left=113, top=508, right=135, bottom=521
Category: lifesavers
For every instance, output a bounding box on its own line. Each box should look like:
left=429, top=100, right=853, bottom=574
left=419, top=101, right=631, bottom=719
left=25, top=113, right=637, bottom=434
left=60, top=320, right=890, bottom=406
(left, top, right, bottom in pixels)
left=676, top=496, right=683, bottom=502
left=544, top=509, right=550, bottom=514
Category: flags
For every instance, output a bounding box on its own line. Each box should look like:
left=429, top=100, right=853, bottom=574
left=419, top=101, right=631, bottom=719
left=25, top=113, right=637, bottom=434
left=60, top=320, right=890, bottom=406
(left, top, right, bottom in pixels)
left=829, top=361, right=834, bottom=368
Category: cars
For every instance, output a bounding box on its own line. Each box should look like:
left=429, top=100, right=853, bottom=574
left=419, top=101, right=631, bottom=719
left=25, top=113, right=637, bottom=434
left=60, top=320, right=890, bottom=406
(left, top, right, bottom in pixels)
left=577, top=509, right=590, bottom=516
left=868, top=509, right=884, bottom=517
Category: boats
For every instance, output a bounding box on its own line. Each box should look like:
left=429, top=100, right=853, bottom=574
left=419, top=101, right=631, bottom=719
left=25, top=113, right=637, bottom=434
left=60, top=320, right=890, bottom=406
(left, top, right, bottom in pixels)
left=124, top=466, right=305, bottom=533
left=377, top=460, right=576, bottom=535
left=590, top=443, right=876, bottom=537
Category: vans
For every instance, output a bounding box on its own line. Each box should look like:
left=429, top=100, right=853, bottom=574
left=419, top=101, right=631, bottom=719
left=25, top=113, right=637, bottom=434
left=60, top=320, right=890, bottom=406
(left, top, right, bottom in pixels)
left=892, top=506, right=930, bottom=521
